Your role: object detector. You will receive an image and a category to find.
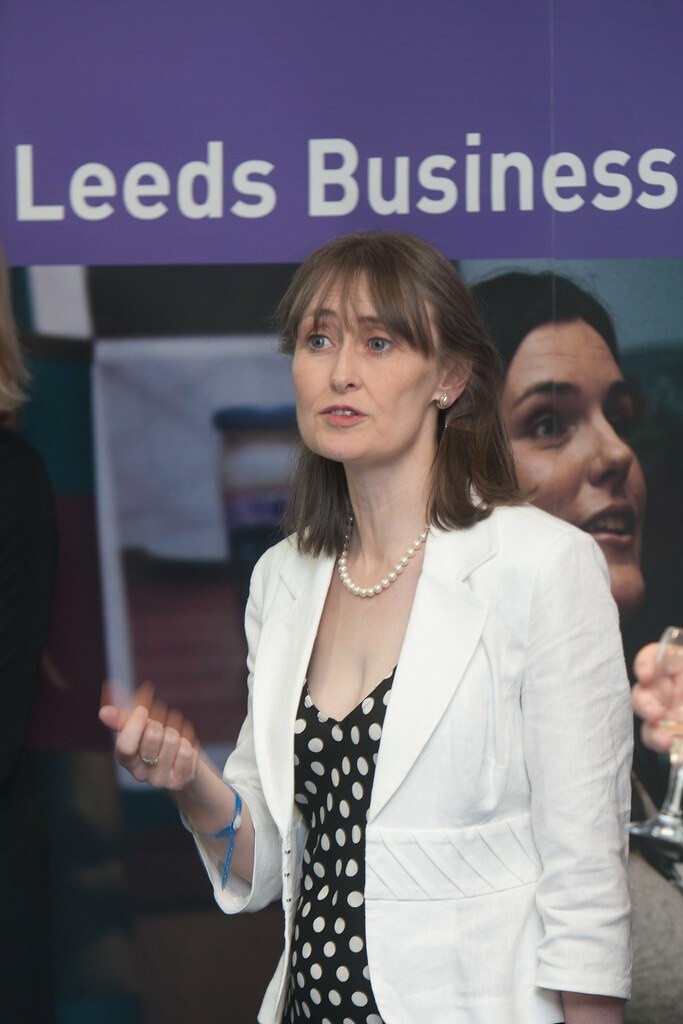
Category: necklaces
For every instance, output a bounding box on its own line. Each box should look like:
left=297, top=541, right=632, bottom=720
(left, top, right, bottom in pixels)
left=336, top=517, right=430, bottom=599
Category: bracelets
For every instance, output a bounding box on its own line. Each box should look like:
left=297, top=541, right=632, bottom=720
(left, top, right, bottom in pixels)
left=179, top=781, right=242, bottom=889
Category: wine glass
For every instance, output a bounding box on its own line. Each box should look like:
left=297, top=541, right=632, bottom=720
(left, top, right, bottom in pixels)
left=628, top=625, right=683, bottom=847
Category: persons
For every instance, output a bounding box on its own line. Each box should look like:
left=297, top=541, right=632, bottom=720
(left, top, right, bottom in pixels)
left=633, top=641, right=683, bottom=765
left=97, top=231, right=634, bottom=1024
left=469, top=270, right=683, bottom=1024
left=0, top=247, right=55, bottom=1024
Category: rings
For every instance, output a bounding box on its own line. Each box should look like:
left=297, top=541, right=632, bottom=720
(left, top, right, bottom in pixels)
left=139, top=753, right=158, bottom=766
